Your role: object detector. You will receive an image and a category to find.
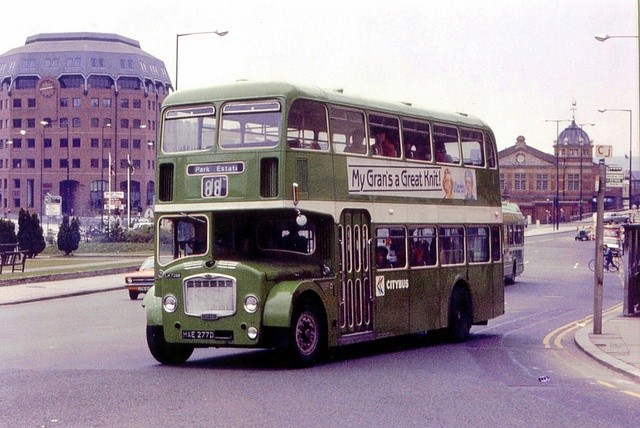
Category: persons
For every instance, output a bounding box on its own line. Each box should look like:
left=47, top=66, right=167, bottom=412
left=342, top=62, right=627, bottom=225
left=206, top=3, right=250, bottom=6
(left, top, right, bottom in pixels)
left=372, top=129, right=395, bottom=156
left=464, top=170, right=476, bottom=200
left=443, top=168, right=455, bottom=200
left=604, top=244, right=620, bottom=271
left=344, top=129, right=367, bottom=154
left=415, top=240, right=429, bottom=266
left=436, top=140, right=453, bottom=163
left=375, top=245, right=393, bottom=267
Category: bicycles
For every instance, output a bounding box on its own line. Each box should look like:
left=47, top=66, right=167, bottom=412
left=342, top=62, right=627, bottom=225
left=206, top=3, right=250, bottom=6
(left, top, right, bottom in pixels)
left=587, top=257, right=623, bottom=274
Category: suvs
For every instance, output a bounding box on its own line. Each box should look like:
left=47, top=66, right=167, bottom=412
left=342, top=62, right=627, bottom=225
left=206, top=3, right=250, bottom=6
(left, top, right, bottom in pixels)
left=576, top=220, right=594, bottom=241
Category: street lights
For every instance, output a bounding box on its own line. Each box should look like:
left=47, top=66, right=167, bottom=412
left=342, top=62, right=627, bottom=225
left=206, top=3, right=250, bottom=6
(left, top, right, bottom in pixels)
left=40, top=119, right=70, bottom=232
left=129, top=125, right=146, bottom=228
left=101, top=123, right=112, bottom=227
left=597, top=108, right=633, bottom=217
left=19, top=128, right=44, bottom=227
left=545, top=119, right=575, bottom=229
left=175, top=29, right=230, bottom=91
left=578, top=121, right=595, bottom=222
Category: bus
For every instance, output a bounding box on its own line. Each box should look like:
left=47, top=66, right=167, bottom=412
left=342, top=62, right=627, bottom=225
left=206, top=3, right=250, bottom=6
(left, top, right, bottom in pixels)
left=502, top=196, right=527, bottom=287
left=144, top=78, right=501, bottom=363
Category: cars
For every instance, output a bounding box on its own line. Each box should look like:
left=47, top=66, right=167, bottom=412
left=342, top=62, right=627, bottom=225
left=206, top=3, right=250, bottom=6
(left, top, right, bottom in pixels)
left=125, top=254, right=171, bottom=299
left=603, top=212, right=631, bottom=224
left=603, top=237, right=622, bottom=257
